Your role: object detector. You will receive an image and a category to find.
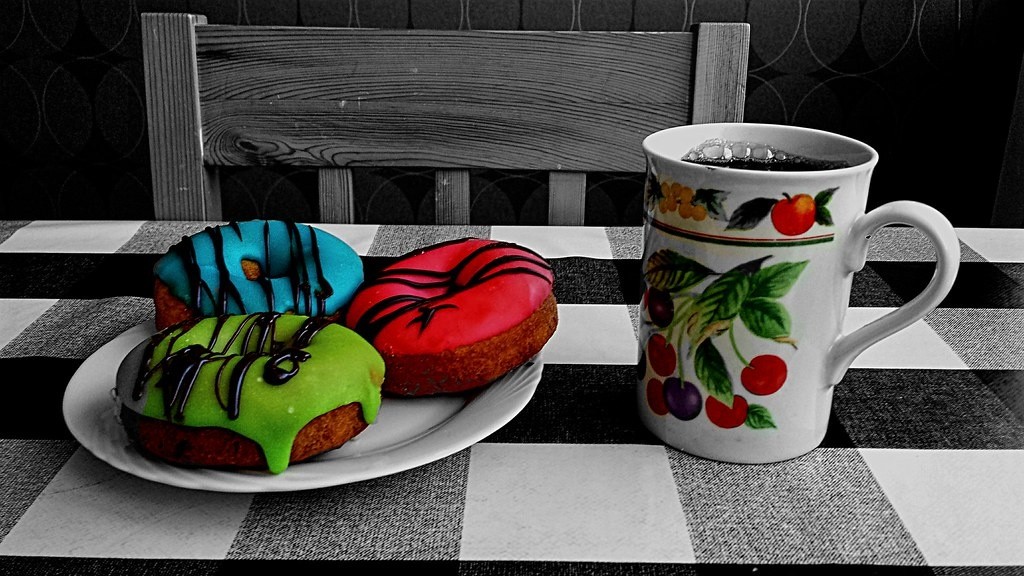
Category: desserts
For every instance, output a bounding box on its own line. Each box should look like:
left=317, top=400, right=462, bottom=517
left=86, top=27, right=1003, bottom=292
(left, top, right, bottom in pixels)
left=345, top=235, right=558, bottom=396
left=109, top=314, right=387, bottom=478
left=150, top=215, right=368, bottom=334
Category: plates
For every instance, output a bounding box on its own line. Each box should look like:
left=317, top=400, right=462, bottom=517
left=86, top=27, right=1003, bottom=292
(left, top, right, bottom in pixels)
left=63, top=319, right=543, bottom=493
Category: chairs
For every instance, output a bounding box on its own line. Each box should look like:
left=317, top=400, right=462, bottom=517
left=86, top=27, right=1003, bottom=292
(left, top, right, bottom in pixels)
left=140, top=12, right=751, bottom=224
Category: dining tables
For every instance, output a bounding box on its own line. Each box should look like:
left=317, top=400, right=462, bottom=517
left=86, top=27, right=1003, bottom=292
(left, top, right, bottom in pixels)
left=0, top=220, right=1020, bottom=576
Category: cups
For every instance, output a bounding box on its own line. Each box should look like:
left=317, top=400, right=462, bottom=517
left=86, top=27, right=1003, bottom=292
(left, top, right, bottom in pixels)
left=638, top=123, right=961, bottom=463
